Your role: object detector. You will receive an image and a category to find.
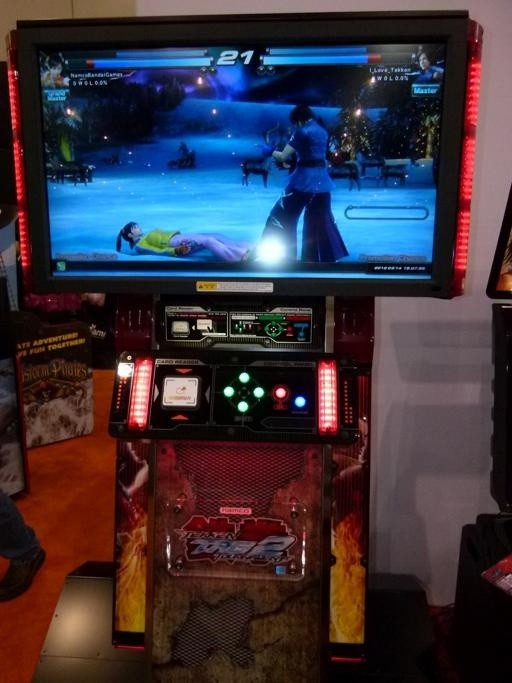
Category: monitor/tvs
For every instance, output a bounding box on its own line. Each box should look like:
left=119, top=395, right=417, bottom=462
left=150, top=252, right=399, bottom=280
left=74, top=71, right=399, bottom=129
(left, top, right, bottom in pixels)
left=6, top=9, right=484, bottom=300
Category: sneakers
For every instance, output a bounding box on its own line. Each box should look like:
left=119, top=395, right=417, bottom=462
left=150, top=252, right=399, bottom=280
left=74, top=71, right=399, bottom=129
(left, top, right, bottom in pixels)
left=0, top=550, right=46, bottom=602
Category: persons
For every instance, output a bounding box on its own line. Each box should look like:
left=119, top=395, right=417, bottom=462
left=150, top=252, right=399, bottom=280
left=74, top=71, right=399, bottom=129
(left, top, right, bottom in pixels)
left=2, top=489, right=48, bottom=606
left=255, top=101, right=350, bottom=264
left=412, top=45, right=443, bottom=84
left=114, top=219, right=252, bottom=264
left=42, top=53, right=70, bottom=89
